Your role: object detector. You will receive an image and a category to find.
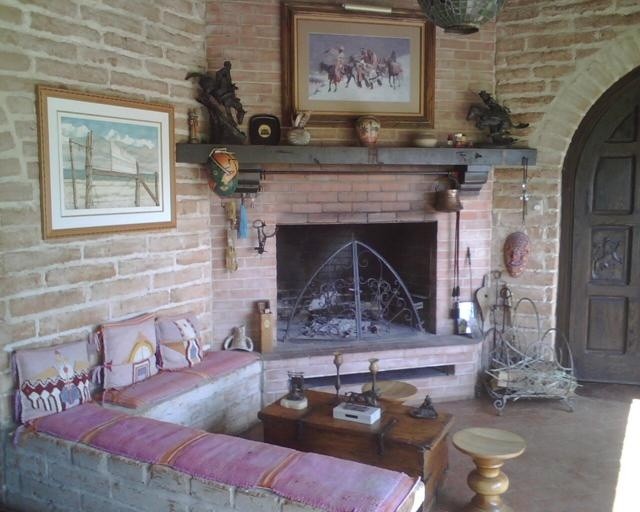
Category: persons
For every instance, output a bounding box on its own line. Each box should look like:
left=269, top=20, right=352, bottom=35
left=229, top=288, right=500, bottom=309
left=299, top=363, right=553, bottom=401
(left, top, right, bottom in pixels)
left=213, top=61, right=246, bottom=126
left=333, top=47, right=345, bottom=82
left=389, top=50, right=398, bottom=62
left=359, top=48, right=369, bottom=63
left=367, top=49, right=379, bottom=66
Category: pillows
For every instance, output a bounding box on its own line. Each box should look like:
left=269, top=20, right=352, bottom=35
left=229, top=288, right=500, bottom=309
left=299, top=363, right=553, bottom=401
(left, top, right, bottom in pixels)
left=99, top=313, right=160, bottom=389
left=154, top=310, right=204, bottom=370
left=11, top=339, right=92, bottom=424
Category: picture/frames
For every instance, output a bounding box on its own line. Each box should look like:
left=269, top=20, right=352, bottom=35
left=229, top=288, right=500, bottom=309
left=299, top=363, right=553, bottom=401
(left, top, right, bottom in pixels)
left=281, top=0, right=436, bottom=130
left=35, top=83, right=176, bottom=240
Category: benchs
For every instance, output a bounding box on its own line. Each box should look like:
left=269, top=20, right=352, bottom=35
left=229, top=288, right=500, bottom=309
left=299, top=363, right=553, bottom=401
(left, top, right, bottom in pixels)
left=5, top=400, right=427, bottom=512
left=93, top=347, right=262, bottom=435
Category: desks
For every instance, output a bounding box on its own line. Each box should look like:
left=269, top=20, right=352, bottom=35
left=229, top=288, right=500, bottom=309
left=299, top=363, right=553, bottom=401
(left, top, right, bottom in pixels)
left=258, top=390, right=456, bottom=512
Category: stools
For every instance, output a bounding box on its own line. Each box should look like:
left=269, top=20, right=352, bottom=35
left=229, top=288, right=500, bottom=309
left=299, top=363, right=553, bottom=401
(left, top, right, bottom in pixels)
left=454, top=426, right=525, bottom=512
left=362, top=381, right=417, bottom=404
